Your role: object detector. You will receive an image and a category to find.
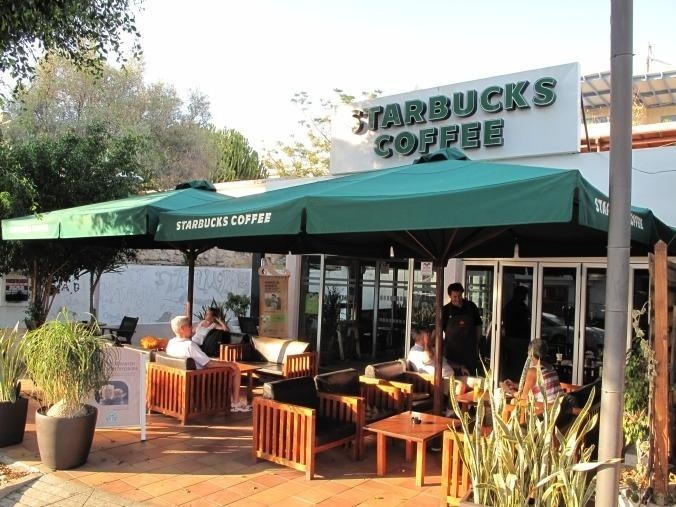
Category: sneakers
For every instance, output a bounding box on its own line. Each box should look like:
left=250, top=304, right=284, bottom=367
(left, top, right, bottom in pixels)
left=230, top=402, right=253, bottom=413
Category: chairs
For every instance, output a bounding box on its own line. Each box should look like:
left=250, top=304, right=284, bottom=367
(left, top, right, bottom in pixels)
left=100, top=316, right=138, bottom=345
left=246, top=360, right=601, bottom=507
left=141, top=328, right=318, bottom=427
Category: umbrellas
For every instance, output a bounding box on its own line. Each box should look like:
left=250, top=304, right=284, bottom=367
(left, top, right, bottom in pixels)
left=153, top=145, right=675, bottom=419
left=1, top=180, right=235, bottom=325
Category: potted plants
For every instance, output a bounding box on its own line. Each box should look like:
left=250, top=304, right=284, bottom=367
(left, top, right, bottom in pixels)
left=16, top=306, right=121, bottom=470
left=0, top=321, right=30, bottom=448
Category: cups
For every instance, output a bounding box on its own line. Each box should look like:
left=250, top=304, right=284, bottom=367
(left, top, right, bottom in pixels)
left=474, top=379, right=482, bottom=392
left=99, top=384, right=114, bottom=400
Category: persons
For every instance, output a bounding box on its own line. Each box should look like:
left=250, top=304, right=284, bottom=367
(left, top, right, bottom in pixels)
left=166, top=315, right=253, bottom=413
left=503, top=285, right=531, bottom=345
left=431, top=283, right=483, bottom=376
left=407, top=325, right=474, bottom=418
left=503, top=339, right=572, bottom=426
left=191, top=307, right=230, bottom=345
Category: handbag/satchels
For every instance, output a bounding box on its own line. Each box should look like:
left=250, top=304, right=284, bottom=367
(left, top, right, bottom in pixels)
left=203, top=329, right=231, bottom=357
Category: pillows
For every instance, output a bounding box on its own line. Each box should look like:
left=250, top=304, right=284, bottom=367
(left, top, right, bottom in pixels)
left=123, top=345, right=155, bottom=362
